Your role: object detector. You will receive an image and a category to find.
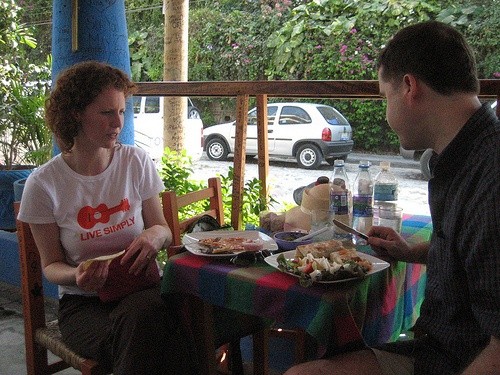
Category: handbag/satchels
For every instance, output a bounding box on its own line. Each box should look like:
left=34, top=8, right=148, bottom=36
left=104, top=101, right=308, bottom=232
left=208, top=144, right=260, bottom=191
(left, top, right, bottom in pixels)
left=98, top=251, right=160, bottom=302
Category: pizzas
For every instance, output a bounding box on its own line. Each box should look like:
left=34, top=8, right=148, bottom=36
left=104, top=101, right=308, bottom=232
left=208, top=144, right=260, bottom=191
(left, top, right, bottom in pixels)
left=200, top=237, right=235, bottom=253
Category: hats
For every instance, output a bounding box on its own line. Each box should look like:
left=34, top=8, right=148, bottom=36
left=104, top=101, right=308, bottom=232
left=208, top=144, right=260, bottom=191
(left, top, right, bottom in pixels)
left=293, top=176, right=346, bottom=206
left=285, top=184, right=354, bottom=234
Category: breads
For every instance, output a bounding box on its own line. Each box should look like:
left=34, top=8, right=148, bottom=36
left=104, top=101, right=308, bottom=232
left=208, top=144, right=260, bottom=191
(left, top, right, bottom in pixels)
left=296, top=240, right=344, bottom=258
left=329, top=250, right=361, bottom=262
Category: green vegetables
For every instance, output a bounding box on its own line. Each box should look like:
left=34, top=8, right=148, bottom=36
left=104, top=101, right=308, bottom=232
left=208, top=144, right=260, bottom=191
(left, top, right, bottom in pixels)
left=276, top=253, right=368, bottom=287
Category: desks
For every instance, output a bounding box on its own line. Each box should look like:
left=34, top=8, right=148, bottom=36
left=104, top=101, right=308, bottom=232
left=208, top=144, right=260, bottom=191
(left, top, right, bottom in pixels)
left=161, top=212, right=434, bottom=375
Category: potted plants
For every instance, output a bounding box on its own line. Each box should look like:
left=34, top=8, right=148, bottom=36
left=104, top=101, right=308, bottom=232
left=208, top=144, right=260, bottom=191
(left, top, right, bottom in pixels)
left=0, top=0, right=68, bottom=232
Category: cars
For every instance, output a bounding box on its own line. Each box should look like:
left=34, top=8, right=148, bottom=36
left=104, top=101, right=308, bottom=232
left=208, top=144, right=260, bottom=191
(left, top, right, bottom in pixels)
left=132, top=95, right=204, bottom=161
left=203, top=103, right=355, bottom=170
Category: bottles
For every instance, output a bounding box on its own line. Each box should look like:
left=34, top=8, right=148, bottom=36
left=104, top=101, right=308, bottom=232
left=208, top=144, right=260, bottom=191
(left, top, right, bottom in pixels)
left=352, top=160, right=374, bottom=246
left=372, top=162, right=398, bottom=226
left=328, top=159, right=353, bottom=242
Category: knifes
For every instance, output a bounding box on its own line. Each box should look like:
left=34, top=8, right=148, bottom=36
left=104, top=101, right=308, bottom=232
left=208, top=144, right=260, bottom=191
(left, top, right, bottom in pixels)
left=332, top=218, right=369, bottom=240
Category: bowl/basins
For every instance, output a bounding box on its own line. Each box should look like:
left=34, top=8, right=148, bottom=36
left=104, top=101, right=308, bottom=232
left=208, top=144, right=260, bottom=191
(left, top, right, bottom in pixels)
left=273, top=231, right=313, bottom=251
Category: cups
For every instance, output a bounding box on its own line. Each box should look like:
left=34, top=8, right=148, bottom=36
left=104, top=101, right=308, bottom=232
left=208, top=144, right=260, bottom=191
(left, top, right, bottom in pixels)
left=259, top=211, right=286, bottom=232
left=310, top=208, right=335, bottom=242
left=379, top=205, right=403, bottom=234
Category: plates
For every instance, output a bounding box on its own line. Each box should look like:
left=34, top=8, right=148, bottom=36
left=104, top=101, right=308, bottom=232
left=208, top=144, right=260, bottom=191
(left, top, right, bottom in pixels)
left=183, top=231, right=279, bottom=256
left=264, top=250, right=391, bottom=284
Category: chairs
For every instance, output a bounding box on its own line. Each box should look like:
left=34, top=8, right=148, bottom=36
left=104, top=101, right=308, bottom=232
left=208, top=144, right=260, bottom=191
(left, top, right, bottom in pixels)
left=16, top=176, right=307, bottom=375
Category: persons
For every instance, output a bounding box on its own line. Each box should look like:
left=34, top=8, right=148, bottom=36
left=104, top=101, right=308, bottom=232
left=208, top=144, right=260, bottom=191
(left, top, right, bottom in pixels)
left=16, top=61, right=190, bottom=375
left=283, top=20, right=500, bottom=375
